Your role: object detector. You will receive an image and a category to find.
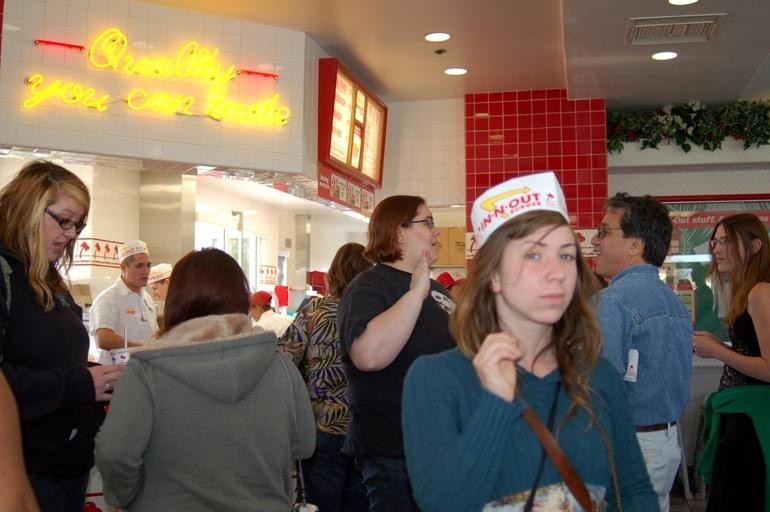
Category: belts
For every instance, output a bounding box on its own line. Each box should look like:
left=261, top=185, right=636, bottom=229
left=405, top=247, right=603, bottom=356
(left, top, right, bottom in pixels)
left=633, top=420, right=678, bottom=434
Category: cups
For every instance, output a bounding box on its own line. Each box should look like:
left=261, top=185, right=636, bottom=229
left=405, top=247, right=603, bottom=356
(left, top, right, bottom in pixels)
left=109, top=347, right=140, bottom=366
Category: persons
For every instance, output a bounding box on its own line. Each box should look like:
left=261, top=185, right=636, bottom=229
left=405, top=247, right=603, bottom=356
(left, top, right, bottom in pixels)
left=0, top=159, right=121, bottom=512
left=584, top=191, right=693, bottom=512
left=0, top=371, right=40, bottom=512
left=401, top=171, right=662, bottom=512
left=146, top=264, right=174, bottom=301
left=94, top=247, right=316, bottom=512
left=690, top=266, right=714, bottom=331
left=250, top=290, right=296, bottom=338
left=88, top=241, right=158, bottom=364
left=693, top=214, right=769, bottom=512
left=339, top=194, right=458, bottom=511
left=278, top=242, right=373, bottom=512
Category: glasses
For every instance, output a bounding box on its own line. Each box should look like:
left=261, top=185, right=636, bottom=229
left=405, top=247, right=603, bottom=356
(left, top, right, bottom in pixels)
left=42, top=205, right=87, bottom=235
left=710, top=236, right=750, bottom=247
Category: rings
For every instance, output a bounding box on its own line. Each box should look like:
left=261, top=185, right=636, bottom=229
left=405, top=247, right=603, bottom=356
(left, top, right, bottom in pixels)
left=105, top=383, right=109, bottom=391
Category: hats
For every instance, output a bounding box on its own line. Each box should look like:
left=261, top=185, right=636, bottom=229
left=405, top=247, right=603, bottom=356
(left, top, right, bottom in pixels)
left=434, top=269, right=466, bottom=287
left=468, top=171, right=571, bottom=249
left=146, top=261, right=177, bottom=286
left=114, top=240, right=151, bottom=263
left=250, top=289, right=272, bottom=305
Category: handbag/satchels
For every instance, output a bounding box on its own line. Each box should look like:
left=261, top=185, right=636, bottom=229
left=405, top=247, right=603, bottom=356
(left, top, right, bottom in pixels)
left=294, top=501, right=319, bottom=511
